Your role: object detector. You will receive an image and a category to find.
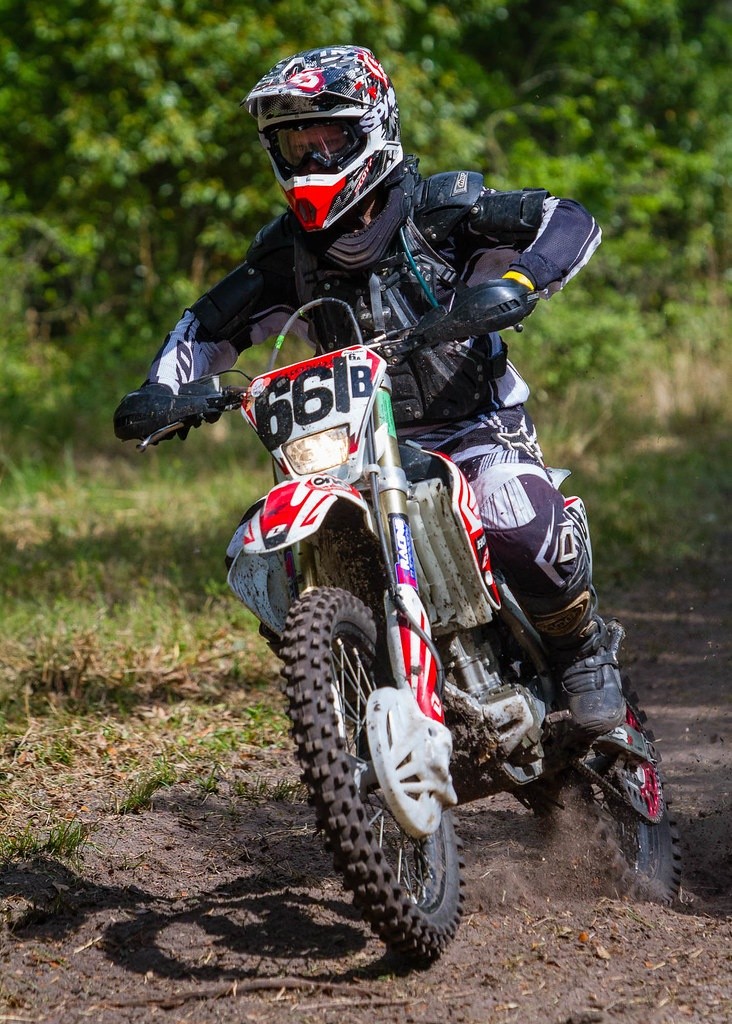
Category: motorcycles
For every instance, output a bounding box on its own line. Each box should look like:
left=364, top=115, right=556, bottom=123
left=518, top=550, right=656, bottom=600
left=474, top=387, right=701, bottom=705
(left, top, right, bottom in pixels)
left=134, top=279, right=677, bottom=965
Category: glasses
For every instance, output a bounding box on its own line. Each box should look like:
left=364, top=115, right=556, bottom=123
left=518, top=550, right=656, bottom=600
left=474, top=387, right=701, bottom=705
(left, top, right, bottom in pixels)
left=265, top=122, right=367, bottom=172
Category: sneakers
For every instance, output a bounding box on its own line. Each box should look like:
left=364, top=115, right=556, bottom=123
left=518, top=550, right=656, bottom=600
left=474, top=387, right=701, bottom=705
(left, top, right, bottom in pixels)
left=557, top=614, right=626, bottom=735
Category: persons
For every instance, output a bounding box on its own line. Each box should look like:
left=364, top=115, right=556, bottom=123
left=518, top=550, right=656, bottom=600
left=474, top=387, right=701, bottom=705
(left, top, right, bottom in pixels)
left=113, top=44, right=628, bottom=734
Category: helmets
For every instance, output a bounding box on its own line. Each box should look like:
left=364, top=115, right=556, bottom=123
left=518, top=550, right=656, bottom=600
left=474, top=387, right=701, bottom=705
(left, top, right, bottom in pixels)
left=238, top=44, right=405, bottom=232
